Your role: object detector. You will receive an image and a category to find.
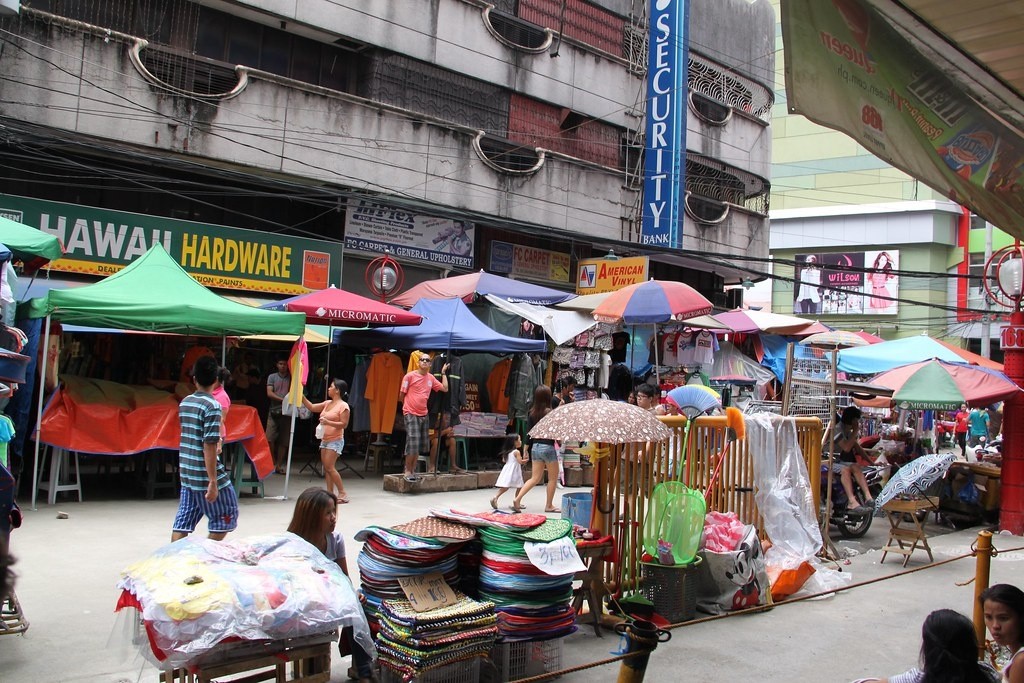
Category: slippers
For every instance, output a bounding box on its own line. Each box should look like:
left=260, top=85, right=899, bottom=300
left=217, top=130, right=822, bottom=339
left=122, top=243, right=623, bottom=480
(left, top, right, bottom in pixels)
left=449, top=468, right=465, bottom=474
left=427, top=468, right=440, bottom=474
left=404, top=473, right=422, bottom=482
left=863, top=499, right=875, bottom=507
left=508, top=506, right=521, bottom=513
left=846, top=507, right=873, bottom=514
left=545, top=507, right=561, bottom=513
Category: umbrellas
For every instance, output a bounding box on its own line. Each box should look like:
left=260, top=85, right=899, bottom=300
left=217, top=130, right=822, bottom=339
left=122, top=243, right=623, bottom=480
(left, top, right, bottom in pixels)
left=590, top=276, right=714, bottom=384
left=386, top=268, right=579, bottom=308
left=527, top=398, right=674, bottom=514
left=554, top=291, right=735, bottom=333
left=682, top=306, right=1024, bottom=417
left=872, top=452, right=958, bottom=529
left=256, top=284, right=423, bottom=479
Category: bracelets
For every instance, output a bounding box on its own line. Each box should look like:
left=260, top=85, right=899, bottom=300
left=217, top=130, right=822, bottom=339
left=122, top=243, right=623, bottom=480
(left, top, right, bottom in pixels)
left=441, top=373, right=446, bottom=376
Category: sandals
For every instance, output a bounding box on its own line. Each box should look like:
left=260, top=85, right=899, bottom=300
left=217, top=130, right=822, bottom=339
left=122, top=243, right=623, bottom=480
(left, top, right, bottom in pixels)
left=275, top=469, right=286, bottom=475
left=336, top=495, right=349, bottom=504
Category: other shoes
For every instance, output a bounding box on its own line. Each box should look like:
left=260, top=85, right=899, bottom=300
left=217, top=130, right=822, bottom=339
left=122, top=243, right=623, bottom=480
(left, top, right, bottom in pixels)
left=489, top=496, right=498, bottom=509
left=619, top=512, right=632, bottom=520
left=520, top=504, right=527, bottom=508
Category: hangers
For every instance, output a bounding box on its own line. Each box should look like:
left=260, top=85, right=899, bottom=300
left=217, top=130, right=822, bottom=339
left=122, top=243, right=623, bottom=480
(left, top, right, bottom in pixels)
left=658, top=325, right=709, bottom=335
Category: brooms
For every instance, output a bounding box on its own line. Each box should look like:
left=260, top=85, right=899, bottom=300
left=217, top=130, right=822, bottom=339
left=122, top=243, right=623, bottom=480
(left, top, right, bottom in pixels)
left=689, top=406, right=747, bottom=538
left=665, top=383, right=721, bottom=543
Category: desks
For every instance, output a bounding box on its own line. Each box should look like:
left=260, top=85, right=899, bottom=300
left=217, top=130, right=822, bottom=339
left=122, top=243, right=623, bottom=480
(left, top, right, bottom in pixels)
left=447, top=434, right=516, bottom=472
left=160, top=642, right=332, bottom=683
left=571, top=546, right=615, bottom=638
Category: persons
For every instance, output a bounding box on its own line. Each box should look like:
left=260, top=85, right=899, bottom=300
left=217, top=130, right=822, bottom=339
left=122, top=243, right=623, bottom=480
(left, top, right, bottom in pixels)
left=620, top=383, right=708, bottom=498
left=820, top=406, right=876, bottom=515
left=607, top=323, right=631, bottom=365
left=977, top=584, right=1024, bottom=683
left=906, top=403, right=990, bottom=461
left=796, top=255, right=820, bottom=315
left=851, top=609, right=993, bottom=683
left=286, top=486, right=372, bottom=679
left=170, top=353, right=240, bottom=542
left=866, top=252, right=896, bottom=308
left=824, top=286, right=861, bottom=310
left=491, top=375, right=578, bottom=513
left=762, top=378, right=783, bottom=404
left=437, top=220, right=472, bottom=256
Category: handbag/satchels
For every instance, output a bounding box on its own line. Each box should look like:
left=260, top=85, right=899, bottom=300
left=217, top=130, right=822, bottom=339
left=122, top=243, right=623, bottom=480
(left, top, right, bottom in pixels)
left=696, top=523, right=774, bottom=615
left=282, top=393, right=298, bottom=418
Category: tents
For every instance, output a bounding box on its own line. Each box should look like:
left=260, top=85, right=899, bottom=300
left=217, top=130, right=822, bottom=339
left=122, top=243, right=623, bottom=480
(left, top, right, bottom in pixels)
left=15, top=241, right=306, bottom=511
left=331, top=295, right=547, bottom=358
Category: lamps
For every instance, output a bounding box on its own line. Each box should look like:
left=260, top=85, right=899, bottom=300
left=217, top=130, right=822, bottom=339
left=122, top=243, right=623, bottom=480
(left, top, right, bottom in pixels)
left=559, top=106, right=589, bottom=133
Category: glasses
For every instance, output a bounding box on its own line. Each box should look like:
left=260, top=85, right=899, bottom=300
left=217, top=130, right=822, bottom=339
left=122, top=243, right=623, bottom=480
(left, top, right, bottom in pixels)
left=636, top=395, right=648, bottom=399
left=419, top=358, right=431, bottom=362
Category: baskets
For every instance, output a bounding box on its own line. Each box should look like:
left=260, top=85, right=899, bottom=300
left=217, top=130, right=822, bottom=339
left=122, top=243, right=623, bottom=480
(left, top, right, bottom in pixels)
left=380, top=656, right=479, bottom=683
left=480, top=636, right=563, bottom=683
left=643, top=481, right=706, bottom=564
left=639, top=555, right=702, bottom=624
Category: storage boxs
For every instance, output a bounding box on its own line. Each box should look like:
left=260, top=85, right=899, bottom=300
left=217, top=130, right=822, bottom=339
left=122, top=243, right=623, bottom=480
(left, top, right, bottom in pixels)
left=481, top=633, right=570, bottom=683
left=378, top=650, right=480, bottom=683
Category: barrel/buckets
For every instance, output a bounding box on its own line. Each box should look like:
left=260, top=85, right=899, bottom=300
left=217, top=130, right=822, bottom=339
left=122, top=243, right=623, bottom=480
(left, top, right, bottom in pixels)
left=560, top=491, right=594, bottom=527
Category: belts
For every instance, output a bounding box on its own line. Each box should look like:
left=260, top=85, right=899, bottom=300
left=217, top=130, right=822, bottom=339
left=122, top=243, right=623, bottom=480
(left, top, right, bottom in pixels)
left=873, top=286, right=885, bottom=288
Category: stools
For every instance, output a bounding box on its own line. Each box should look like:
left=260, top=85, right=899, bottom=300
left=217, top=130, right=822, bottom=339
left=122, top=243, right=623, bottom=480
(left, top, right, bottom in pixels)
left=880, top=506, right=939, bottom=567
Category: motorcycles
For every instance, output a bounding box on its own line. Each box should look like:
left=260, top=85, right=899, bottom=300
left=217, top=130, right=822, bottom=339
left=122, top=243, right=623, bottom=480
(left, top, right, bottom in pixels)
left=821, top=462, right=891, bottom=539
left=975, top=437, right=1002, bottom=466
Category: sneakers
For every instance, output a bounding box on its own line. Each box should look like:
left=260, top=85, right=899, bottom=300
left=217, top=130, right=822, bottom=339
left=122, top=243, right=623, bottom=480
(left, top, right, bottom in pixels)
left=556, top=482, right=564, bottom=489
